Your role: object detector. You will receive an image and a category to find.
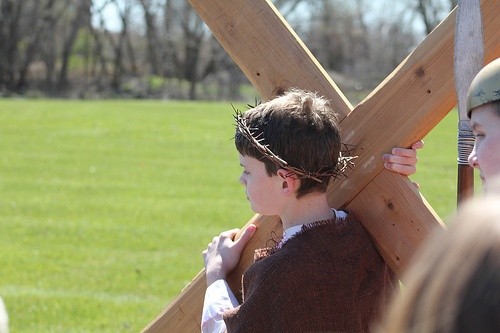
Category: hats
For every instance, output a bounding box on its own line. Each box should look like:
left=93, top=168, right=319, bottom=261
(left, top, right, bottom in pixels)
left=465, top=55, right=500, bottom=117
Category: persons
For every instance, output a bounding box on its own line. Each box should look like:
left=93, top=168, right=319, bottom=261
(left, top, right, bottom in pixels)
left=468, top=55, right=500, bottom=206
left=201, top=87, right=425, bottom=333
